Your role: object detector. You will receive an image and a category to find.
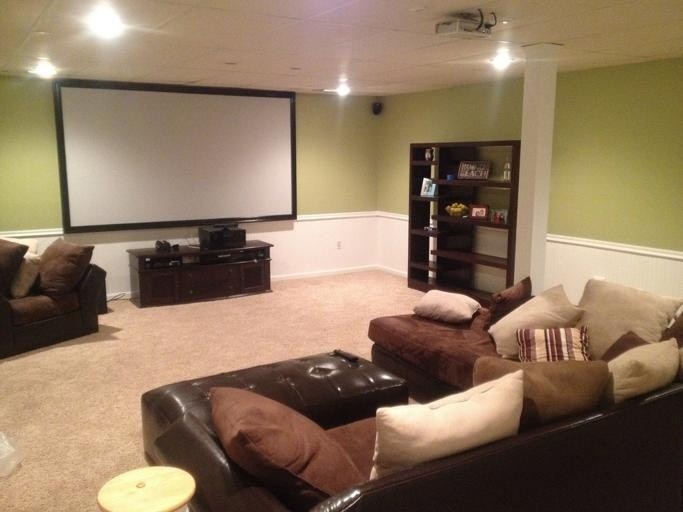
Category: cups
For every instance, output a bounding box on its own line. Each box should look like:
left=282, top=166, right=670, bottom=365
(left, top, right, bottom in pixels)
left=446, top=175, right=454, bottom=180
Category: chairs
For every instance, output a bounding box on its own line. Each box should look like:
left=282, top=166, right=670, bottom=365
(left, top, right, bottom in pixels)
left=0, top=256, right=107, bottom=361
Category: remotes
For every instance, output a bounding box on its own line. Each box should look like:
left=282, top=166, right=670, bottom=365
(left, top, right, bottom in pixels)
left=333, top=349, right=358, bottom=362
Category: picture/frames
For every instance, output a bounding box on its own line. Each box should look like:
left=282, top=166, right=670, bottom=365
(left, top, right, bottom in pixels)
left=468, top=203, right=489, bottom=222
left=490, top=208, right=508, bottom=225
left=457, top=159, right=491, bottom=180
left=421, top=175, right=437, bottom=197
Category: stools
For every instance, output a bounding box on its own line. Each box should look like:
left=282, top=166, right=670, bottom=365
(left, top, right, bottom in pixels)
left=98, top=466, right=198, bottom=511
left=141, top=349, right=409, bottom=511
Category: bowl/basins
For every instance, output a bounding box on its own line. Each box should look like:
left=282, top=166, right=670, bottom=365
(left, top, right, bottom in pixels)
left=444, top=208, right=465, bottom=217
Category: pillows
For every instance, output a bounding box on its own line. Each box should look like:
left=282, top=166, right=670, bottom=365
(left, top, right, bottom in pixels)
left=487, top=284, right=583, bottom=359
left=12, top=250, right=43, bottom=297
left=514, top=327, right=590, bottom=363
left=368, top=370, right=528, bottom=487
left=472, top=355, right=609, bottom=431
left=209, top=385, right=361, bottom=497
left=39, top=236, right=96, bottom=300
left=483, top=277, right=532, bottom=330
left=414, top=290, right=481, bottom=322
left=608, top=337, right=680, bottom=407
left=0, top=237, right=28, bottom=299
left=600, top=329, right=650, bottom=362
left=577, top=279, right=683, bottom=360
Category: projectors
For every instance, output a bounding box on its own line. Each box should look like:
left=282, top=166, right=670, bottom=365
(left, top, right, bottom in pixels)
left=434, top=19, right=492, bottom=39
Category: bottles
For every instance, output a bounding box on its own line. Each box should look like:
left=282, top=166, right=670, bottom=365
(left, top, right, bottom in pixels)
left=503, top=161, right=510, bottom=180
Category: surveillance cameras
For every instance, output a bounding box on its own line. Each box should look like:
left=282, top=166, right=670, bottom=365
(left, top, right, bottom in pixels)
left=370, top=98, right=384, bottom=114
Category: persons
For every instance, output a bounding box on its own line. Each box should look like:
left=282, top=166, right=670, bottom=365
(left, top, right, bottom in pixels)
left=423, top=161, right=487, bottom=216
left=501, top=215, right=504, bottom=223
left=493, top=212, right=500, bottom=222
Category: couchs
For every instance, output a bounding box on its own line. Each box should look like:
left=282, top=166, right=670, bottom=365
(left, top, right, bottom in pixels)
left=141, top=292, right=683, bottom=511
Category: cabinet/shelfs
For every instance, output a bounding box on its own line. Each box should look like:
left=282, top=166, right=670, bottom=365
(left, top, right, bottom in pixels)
left=125, top=239, right=275, bottom=308
left=408, top=139, right=521, bottom=306
